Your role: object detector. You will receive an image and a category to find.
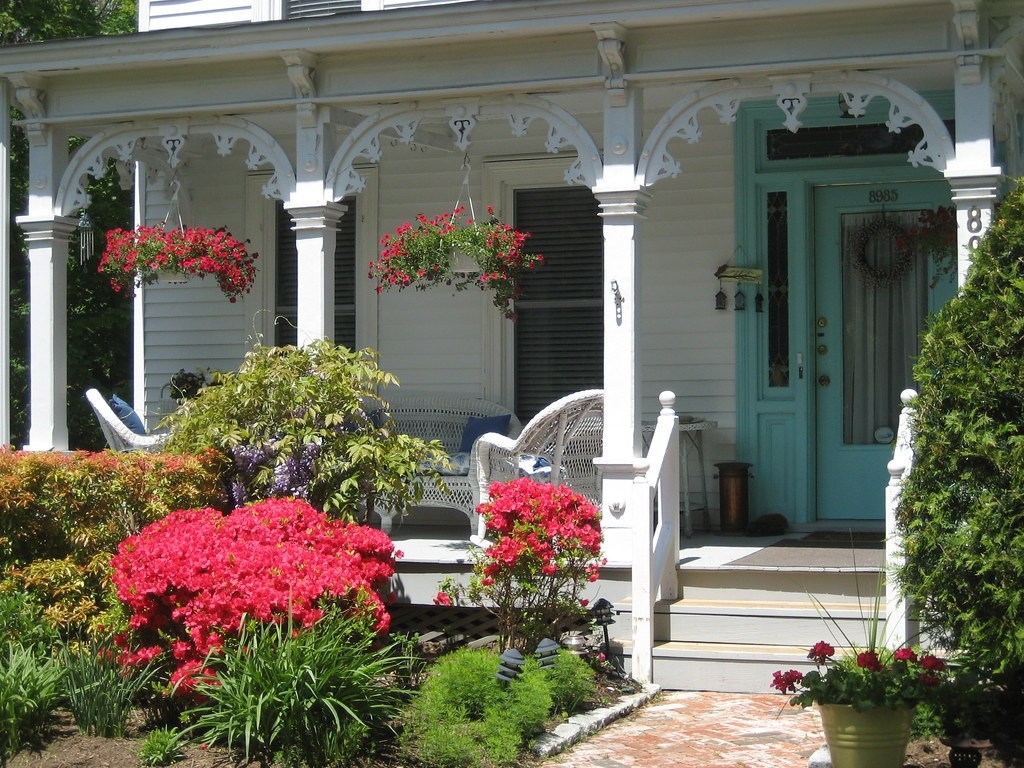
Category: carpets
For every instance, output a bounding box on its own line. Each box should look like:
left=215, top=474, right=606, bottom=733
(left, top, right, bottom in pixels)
left=724, top=528, right=887, bottom=567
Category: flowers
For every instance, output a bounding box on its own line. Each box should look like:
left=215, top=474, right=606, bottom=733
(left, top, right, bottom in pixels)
left=96, top=220, right=260, bottom=306
left=770, top=527, right=960, bottom=708
left=369, top=204, right=544, bottom=323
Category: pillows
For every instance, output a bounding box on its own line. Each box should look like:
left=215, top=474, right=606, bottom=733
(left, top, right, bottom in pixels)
left=343, top=408, right=382, bottom=435
left=459, top=414, right=512, bottom=453
left=108, top=394, right=148, bottom=436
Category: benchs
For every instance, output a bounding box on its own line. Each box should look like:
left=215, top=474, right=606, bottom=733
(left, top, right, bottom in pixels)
left=352, top=397, right=523, bottom=540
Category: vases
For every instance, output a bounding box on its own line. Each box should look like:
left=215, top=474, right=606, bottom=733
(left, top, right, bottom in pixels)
left=449, top=246, right=485, bottom=273
left=155, top=268, right=192, bottom=283
left=813, top=703, right=917, bottom=768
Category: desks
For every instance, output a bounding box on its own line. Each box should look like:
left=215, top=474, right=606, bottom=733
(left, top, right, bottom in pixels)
left=640, top=420, right=717, bottom=537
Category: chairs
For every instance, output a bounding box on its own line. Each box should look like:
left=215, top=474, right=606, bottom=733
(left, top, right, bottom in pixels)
left=469, top=388, right=605, bottom=549
left=84, top=389, right=170, bottom=454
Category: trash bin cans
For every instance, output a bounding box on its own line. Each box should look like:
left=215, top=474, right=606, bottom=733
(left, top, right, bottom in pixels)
left=712, top=461, right=755, bottom=536
